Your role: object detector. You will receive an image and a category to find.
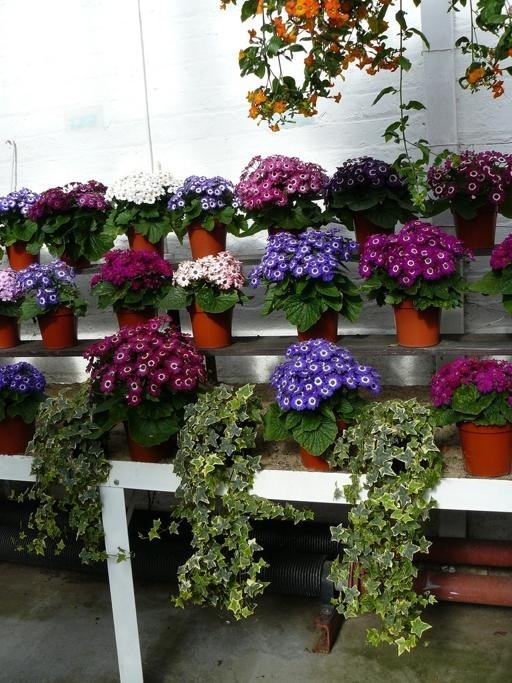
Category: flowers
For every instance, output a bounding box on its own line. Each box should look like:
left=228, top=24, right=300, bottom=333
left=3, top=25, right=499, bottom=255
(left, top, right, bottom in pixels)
left=0, top=266, right=29, bottom=349
left=328, top=157, right=409, bottom=243
left=429, top=356, right=509, bottom=481
left=237, top=154, right=329, bottom=239
left=422, top=149, right=510, bottom=253
left=2, top=360, right=44, bottom=452
left=356, top=221, right=473, bottom=349
left=85, top=315, right=206, bottom=463
left=15, top=260, right=88, bottom=351
left=262, top=337, right=381, bottom=473
left=106, top=169, right=175, bottom=260
left=88, top=245, right=172, bottom=330
left=29, top=180, right=111, bottom=266
left=173, top=251, right=246, bottom=350
left=1, top=188, right=46, bottom=269
left=489, top=235, right=512, bottom=322
left=247, top=231, right=364, bottom=346
left=167, top=174, right=239, bottom=260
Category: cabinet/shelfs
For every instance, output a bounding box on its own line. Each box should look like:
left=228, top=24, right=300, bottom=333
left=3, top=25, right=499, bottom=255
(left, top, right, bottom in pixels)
left=0, top=244, right=509, bottom=682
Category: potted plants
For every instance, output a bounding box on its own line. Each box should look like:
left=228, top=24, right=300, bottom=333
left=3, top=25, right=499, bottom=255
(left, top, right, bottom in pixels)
left=13, top=388, right=122, bottom=565
left=139, top=383, right=314, bottom=625
left=327, top=394, right=440, bottom=656
